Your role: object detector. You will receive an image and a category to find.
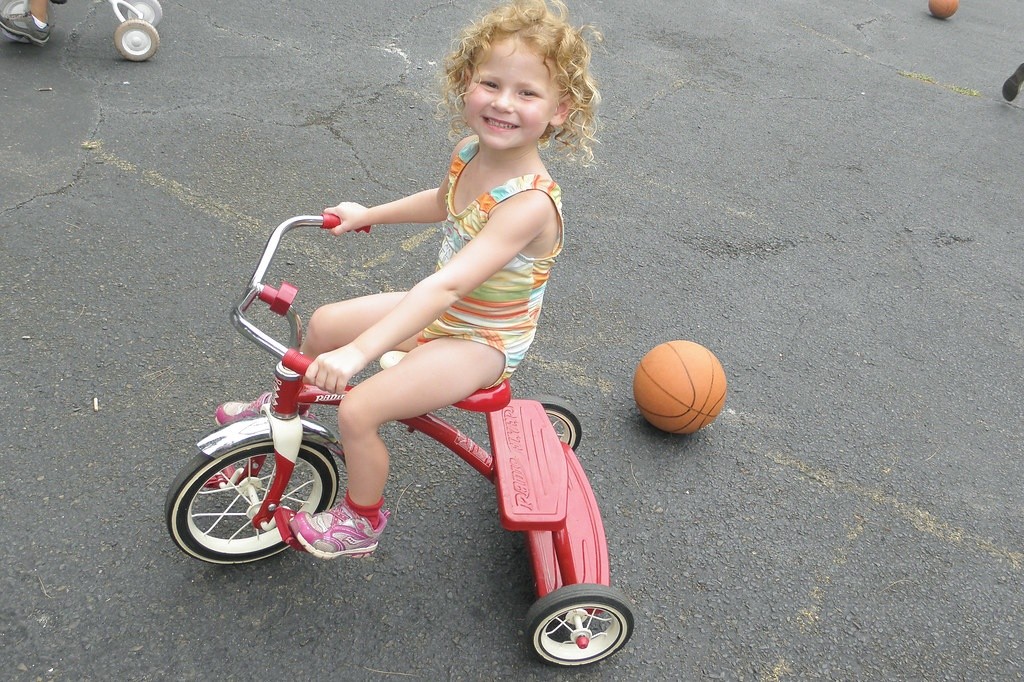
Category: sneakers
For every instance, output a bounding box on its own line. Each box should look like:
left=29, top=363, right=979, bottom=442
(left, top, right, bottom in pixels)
left=215, top=392, right=274, bottom=425
left=0, top=11, right=51, bottom=45
left=288, top=500, right=391, bottom=560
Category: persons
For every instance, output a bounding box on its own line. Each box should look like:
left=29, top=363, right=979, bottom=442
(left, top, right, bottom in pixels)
left=213, top=2, right=603, bottom=563
left=0, top=0, right=52, bottom=45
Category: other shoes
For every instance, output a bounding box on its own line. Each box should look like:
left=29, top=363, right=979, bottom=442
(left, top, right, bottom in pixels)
left=1002, top=62, right=1024, bottom=102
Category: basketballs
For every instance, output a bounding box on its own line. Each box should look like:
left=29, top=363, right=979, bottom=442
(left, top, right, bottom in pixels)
left=928, top=0, right=959, bottom=19
left=633, top=339, right=728, bottom=435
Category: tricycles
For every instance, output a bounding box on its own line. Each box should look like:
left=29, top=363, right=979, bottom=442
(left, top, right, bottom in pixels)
left=0, top=0, right=163, bottom=63
left=162, top=212, right=638, bottom=668
